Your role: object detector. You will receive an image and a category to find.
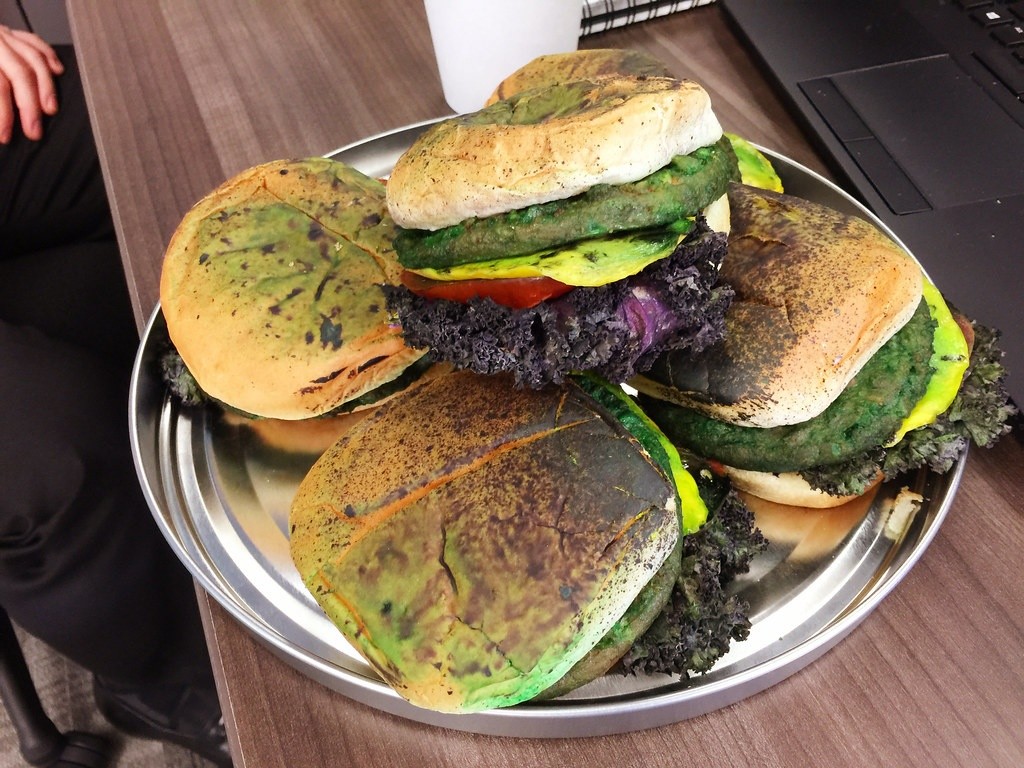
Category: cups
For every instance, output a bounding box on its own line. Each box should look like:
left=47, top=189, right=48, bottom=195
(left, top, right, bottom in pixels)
left=423, top=0, right=584, bottom=120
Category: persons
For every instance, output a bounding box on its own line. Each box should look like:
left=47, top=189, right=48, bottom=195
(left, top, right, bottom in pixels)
left=0, top=22, right=234, bottom=768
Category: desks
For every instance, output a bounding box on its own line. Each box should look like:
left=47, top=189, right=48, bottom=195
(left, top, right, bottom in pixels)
left=66, top=0, right=1024, bottom=768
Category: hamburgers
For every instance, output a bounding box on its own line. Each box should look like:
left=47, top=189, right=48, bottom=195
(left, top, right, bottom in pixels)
left=157, top=51, right=1019, bottom=712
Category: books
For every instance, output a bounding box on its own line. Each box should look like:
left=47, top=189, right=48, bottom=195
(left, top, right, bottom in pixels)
left=579, top=0, right=715, bottom=37
